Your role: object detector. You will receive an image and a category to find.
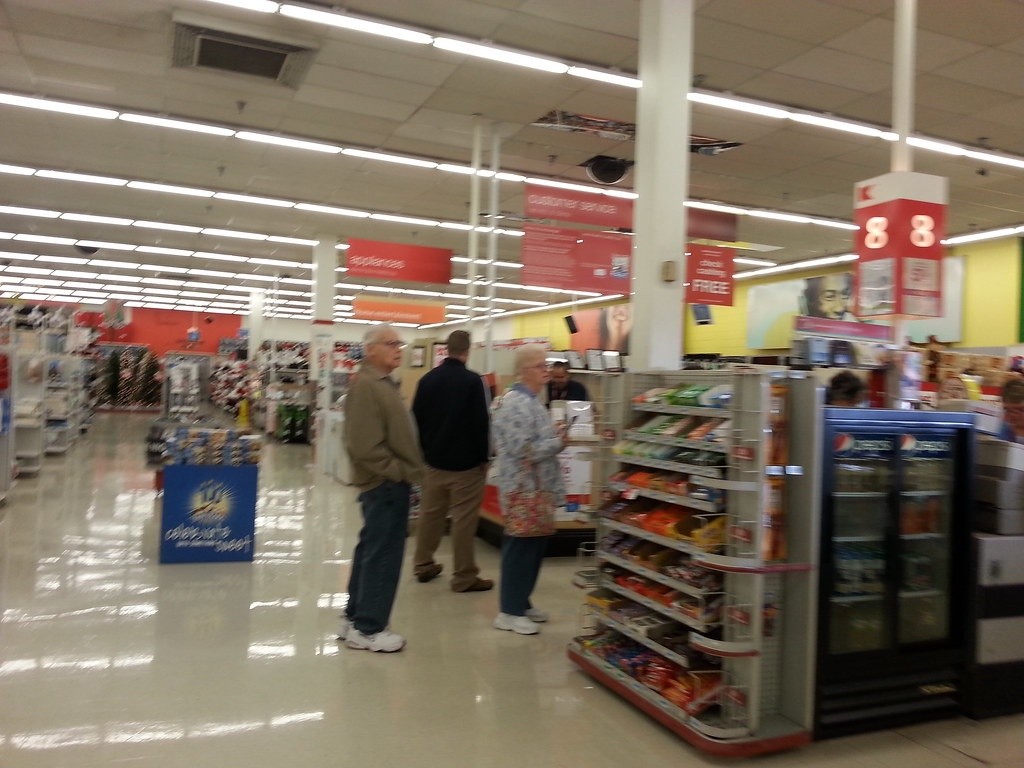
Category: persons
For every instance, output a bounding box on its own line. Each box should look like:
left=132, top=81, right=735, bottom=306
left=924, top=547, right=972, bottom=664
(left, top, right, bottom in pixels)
left=938, top=376, right=969, bottom=402
left=547, top=360, right=591, bottom=407
left=487, top=344, right=574, bottom=635
left=338, top=324, right=426, bottom=654
left=411, top=330, right=495, bottom=592
left=995, top=380, right=1024, bottom=442
left=827, top=370, right=870, bottom=406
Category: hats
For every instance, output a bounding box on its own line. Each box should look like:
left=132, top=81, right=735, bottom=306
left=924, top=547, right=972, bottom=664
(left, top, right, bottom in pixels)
left=1003, top=380, right=1024, bottom=403
left=830, top=370, right=869, bottom=392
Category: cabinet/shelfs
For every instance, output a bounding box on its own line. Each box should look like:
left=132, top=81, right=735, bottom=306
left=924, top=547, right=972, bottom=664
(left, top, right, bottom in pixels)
left=0, top=307, right=366, bottom=564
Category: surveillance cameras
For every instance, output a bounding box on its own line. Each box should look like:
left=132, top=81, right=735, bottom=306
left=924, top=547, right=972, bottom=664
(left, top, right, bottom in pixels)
left=586, top=158, right=627, bottom=185
left=74, top=244, right=99, bottom=258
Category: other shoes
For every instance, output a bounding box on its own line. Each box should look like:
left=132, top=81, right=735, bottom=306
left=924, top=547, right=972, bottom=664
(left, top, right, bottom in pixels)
left=456, top=578, right=493, bottom=593
left=417, top=563, right=442, bottom=582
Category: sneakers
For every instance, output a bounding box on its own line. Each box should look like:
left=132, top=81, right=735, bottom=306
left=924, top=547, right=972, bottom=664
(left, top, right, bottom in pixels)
left=335, top=616, right=356, bottom=638
left=492, top=612, right=542, bottom=634
left=527, top=607, right=548, bottom=622
left=345, top=622, right=406, bottom=652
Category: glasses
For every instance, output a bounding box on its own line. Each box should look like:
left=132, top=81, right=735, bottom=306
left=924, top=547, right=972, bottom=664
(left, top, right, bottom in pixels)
left=522, top=360, right=551, bottom=370
left=373, top=340, right=406, bottom=348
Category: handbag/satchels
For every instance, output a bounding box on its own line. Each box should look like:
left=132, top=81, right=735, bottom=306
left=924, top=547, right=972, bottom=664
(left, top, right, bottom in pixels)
left=504, top=446, right=560, bottom=536
